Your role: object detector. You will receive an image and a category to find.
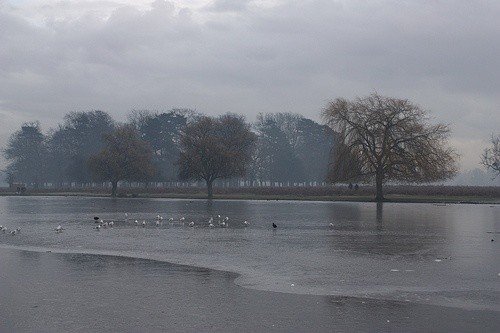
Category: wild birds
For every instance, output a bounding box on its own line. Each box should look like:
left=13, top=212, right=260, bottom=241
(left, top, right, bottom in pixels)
left=244, top=218, right=251, bottom=227
left=329, top=220, right=334, bottom=226
left=273, top=222, right=277, bottom=228
left=0, top=213, right=229, bottom=235
